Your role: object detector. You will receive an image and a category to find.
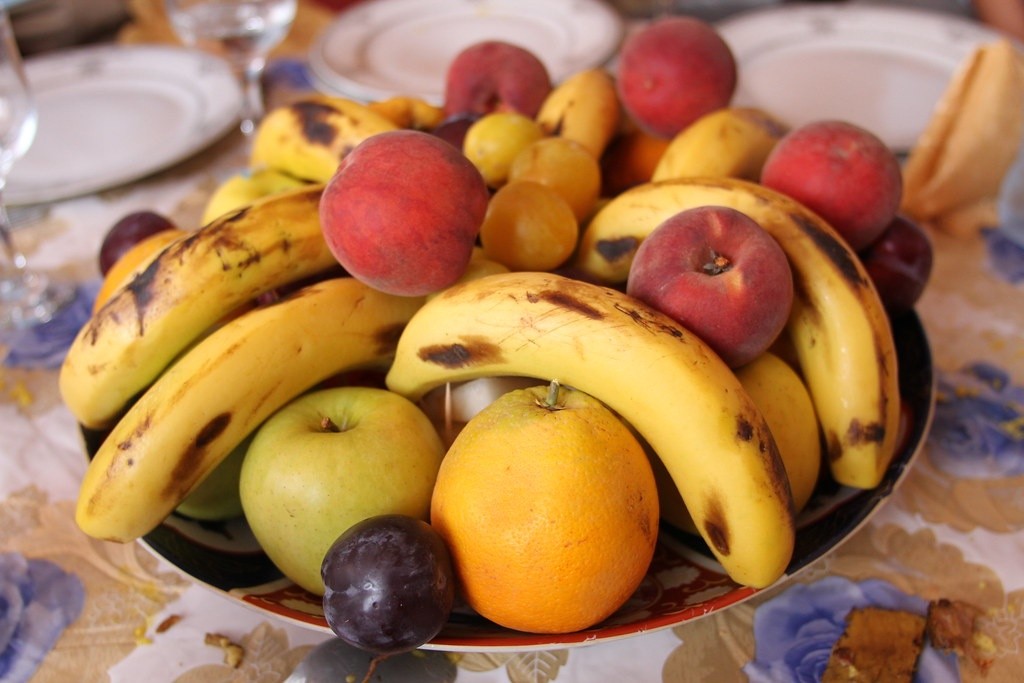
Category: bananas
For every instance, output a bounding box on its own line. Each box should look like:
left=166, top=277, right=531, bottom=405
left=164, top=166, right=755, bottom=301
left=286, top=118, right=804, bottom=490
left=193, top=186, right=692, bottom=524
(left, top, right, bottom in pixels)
left=384, top=271, right=795, bottom=593
left=248, top=95, right=407, bottom=185
left=574, top=178, right=903, bottom=485
left=58, top=184, right=510, bottom=543
left=536, top=67, right=646, bottom=165
left=653, top=111, right=786, bottom=178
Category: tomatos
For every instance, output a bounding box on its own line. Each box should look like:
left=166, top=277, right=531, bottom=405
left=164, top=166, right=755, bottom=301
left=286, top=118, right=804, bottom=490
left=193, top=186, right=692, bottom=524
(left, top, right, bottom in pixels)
left=462, top=112, right=601, bottom=272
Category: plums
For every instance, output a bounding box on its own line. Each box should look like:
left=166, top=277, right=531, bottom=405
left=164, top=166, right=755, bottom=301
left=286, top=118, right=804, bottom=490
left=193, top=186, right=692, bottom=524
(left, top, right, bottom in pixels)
left=856, top=213, right=932, bottom=322
left=99, top=212, right=177, bottom=280
left=318, top=513, right=456, bottom=651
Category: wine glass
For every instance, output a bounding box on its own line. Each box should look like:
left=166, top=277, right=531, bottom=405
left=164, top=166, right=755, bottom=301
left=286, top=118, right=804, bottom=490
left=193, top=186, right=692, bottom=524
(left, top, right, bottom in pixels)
left=164, top=0, right=296, bottom=147
left=2, top=8, right=86, bottom=366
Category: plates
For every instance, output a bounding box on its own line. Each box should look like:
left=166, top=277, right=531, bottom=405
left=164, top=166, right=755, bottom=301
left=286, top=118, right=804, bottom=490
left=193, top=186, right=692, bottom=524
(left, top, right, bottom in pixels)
left=69, top=292, right=937, bottom=655
left=308, top=4, right=618, bottom=104
left=713, top=9, right=1003, bottom=153
left=3, top=46, right=236, bottom=206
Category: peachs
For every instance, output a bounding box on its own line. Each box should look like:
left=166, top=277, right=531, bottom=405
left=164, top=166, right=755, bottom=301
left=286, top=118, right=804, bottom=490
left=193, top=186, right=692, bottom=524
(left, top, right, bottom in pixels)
left=755, top=119, right=903, bottom=255
left=445, top=40, right=553, bottom=123
left=626, top=204, right=794, bottom=373
left=316, top=130, right=488, bottom=300
left=615, top=18, right=737, bottom=140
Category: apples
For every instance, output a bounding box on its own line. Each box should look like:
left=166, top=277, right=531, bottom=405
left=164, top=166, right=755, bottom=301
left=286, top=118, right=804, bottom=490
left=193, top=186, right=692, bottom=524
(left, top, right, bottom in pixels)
left=237, top=385, right=446, bottom=602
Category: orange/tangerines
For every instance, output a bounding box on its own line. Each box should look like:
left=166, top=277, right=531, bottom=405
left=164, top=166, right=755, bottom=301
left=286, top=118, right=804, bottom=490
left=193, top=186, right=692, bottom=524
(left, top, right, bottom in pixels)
left=598, top=133, right=672, bottom=203
left=430, top=377, right=659, bottom=637
